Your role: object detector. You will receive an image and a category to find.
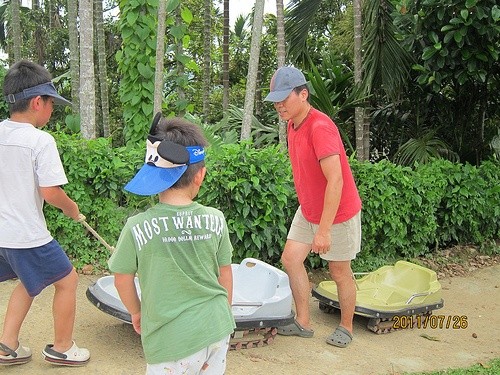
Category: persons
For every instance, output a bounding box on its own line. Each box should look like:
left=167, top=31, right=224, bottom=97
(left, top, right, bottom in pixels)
left=108, top=112, right=236, bottom=375
left=262, top=67, right=361, bottom=348
left=0, top=59, right=92, bottom=368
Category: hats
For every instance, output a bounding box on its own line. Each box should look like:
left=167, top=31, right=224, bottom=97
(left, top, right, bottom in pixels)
left=262, top=66, right=307, bottom=103
left=124, top=136, right=206, bottom=197
left=4, top=81, right=74, bottom=106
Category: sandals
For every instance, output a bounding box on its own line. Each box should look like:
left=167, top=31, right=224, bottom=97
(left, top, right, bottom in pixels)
left=325, top=325, right=354, bottom=348
left=0, top=340, right=33, bottom=366
left=41, top=341, right=91, bottom=366
left=275, top=319, right=314, bottom=338
